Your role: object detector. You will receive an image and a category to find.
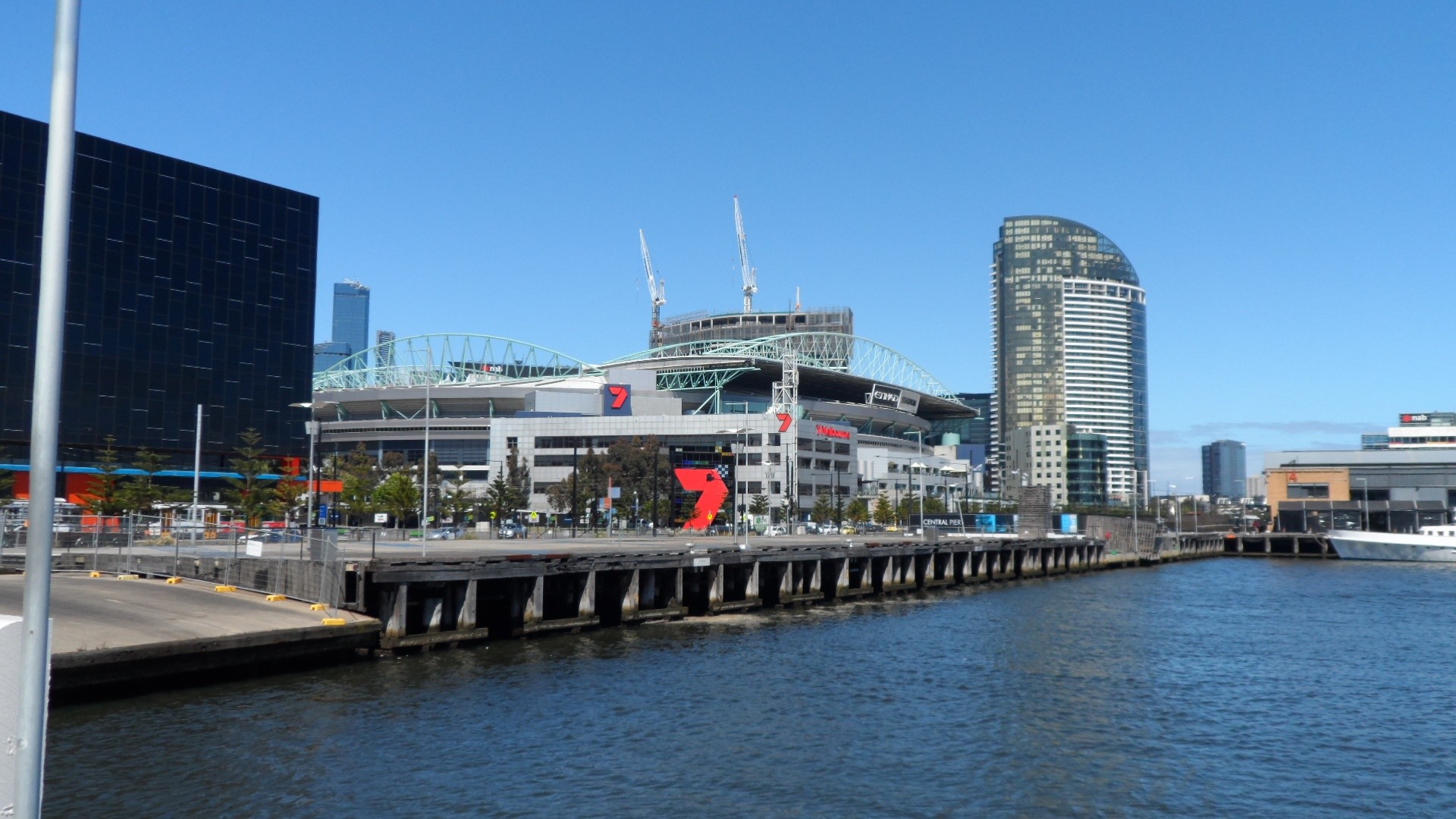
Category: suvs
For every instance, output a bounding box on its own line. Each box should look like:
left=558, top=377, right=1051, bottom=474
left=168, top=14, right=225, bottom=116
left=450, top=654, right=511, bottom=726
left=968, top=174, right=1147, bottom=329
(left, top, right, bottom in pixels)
left=627, top=519, right=651, bottom=531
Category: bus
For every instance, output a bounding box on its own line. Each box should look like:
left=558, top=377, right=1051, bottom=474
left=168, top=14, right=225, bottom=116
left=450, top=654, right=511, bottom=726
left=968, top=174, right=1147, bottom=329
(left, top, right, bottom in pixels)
left=1, top=498, right=608, bottom=539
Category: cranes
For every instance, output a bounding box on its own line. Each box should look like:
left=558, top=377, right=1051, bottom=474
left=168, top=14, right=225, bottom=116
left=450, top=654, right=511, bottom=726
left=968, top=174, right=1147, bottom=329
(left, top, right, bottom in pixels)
left=796, top=286, right=800, bottom=312
left=638, top=229, right=666, bottom=348
left=732, top=195, right=758, bottom=312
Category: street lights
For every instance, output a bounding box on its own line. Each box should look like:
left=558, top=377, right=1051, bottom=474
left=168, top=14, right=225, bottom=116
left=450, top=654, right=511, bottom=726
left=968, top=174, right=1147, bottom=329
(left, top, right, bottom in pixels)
left=1356, top=478, right=1369, bottom=531
left=814, top=466, right=840, bottom=534
left=1235, top=480, right=1246, bottom=532
left=567, top=440, right=576, bottom=538
left=904, top=431, right=924, bottom=541
left=756, top=462, right=784, bottom=535
left=408, top=348, right=431, bottom=554
left=935, top=462, right=969, bottom=513
left=716, top=428, right=761, bottom=542
left=287, top=400, right=340, bottom=549
left=876, top=454, right=946, bottom=520
left=1124, top=476, right=1137, bottom=552
left=766, top=438, right=832, bottom=534
left=726, top=403, right=748, bottom=544
left=483, top=460, right=502, bottom=528
left=1148, top=477, right=1198, bottom=532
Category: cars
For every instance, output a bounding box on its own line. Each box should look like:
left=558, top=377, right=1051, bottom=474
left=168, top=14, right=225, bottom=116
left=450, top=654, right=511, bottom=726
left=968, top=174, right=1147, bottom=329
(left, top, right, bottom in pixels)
left=818, top=525, right=838, bottom=535
left=424, top=527, right=468, bottom=540
left=706, top=522, right=822, bottom=534
left=3, top=526, right=28, bottom=546
left=499, top=523, right=528, bottom=539
left=238, top=528, right=277, bottom=543
left=840, top=524, right=904, bottom=535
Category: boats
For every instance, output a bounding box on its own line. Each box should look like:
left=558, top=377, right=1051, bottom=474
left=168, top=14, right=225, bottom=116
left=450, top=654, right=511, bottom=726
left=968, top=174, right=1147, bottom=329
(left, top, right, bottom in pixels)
left=1316, top=506, right=1456, bottom=563
